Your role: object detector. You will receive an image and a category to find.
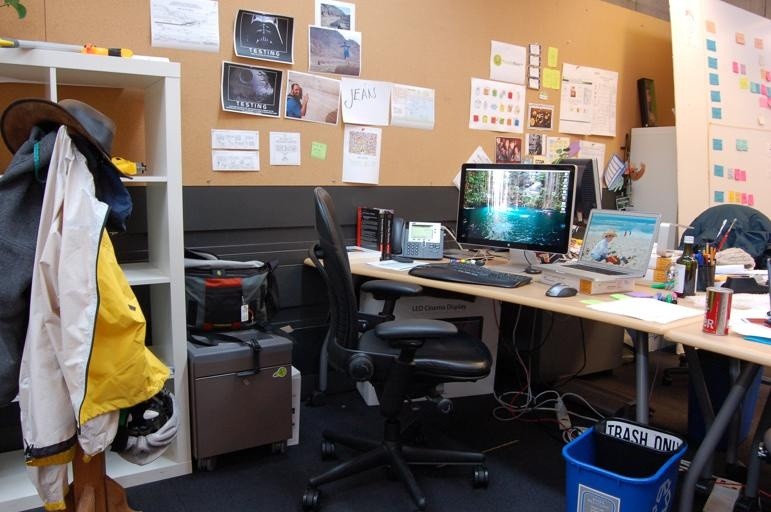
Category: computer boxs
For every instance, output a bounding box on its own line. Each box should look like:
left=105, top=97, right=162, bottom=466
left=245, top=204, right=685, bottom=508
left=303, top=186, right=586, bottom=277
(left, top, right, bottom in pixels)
left=539, top=304, right=625, bottom=384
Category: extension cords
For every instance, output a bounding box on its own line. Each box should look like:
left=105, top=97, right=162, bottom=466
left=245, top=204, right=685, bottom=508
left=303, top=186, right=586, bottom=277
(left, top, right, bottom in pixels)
left=555, top=401, right=571, bottom=429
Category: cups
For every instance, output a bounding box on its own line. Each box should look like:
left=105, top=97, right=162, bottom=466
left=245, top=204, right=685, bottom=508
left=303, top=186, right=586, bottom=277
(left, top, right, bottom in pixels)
left=698, top=258, right=717, bottom=292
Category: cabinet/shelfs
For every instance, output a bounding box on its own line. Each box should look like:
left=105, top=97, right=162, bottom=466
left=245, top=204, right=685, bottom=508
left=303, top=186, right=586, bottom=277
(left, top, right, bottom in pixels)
left=0, top=48, right=192, bottom=511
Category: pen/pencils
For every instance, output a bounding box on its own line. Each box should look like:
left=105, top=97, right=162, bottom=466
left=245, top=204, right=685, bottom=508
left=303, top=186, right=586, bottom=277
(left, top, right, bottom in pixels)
left=715, top=217, right=738, bottom=252
left=694, top=243, right=717, bottom=290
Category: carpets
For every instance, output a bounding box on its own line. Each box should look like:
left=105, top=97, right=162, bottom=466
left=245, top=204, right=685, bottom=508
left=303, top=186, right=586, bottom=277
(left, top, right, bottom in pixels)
left=26, top=386, right=771, bottom=512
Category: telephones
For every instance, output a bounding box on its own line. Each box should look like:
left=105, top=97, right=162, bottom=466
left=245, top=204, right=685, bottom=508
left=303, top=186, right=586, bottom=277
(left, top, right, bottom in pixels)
left=391, top=216, right=444, bottom=260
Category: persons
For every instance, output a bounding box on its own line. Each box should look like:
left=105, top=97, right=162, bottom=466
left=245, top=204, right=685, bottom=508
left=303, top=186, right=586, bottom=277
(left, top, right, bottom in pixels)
left=287, top=83, right=309, bottom=118
left=340, top=42, right=351, bottom=65
left=589, top=227, right=623, bottom=264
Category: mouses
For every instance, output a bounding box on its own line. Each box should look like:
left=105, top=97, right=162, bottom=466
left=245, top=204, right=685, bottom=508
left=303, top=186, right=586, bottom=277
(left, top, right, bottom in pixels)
left=545, top=283, right=577, bottom=297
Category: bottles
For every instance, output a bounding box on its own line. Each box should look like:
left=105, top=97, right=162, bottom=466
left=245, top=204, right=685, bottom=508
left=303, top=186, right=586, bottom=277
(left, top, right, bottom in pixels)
left=674, top=235, right=698, bottom=296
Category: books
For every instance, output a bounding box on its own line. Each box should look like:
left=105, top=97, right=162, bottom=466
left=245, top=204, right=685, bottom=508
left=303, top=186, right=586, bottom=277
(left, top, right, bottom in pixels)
left=357, top=206, right=395, bottom=258
left=533, top=263, right=635, bottom=294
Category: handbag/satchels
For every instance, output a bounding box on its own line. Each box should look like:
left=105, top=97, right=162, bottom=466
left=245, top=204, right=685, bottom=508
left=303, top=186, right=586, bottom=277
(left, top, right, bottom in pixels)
left=184, top=244, right=271, bottom=332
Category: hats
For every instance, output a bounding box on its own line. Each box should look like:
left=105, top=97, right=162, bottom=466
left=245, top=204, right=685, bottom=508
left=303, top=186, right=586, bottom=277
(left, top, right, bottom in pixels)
left=602, top=228, right=618, bottom=238
left=2, top=98, right=133, bottom=180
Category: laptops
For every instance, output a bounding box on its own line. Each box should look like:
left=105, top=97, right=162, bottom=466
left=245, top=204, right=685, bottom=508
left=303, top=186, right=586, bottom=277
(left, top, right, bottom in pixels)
left=532, top=209, right=662, bottom=282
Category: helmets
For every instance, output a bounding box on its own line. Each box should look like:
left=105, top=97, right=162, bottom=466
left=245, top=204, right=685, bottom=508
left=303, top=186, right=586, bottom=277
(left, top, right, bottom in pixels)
left=115, top=388, right=181, bottom=465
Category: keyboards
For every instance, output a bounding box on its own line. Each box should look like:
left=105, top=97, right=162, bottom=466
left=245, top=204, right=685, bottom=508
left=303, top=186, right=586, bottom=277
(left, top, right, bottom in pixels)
left=409, top=262, right=532, bottom=288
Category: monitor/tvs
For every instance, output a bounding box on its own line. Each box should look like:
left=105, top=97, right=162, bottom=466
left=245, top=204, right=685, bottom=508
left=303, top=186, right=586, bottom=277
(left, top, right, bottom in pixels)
left=559, top=158, right=602, bottom=241
left=456, top=163, right=577, bottom=274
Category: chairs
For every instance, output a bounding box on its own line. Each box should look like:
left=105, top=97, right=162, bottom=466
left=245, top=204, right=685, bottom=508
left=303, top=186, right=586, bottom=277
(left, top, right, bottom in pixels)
left=303, top=187, right=490, bottom=511
